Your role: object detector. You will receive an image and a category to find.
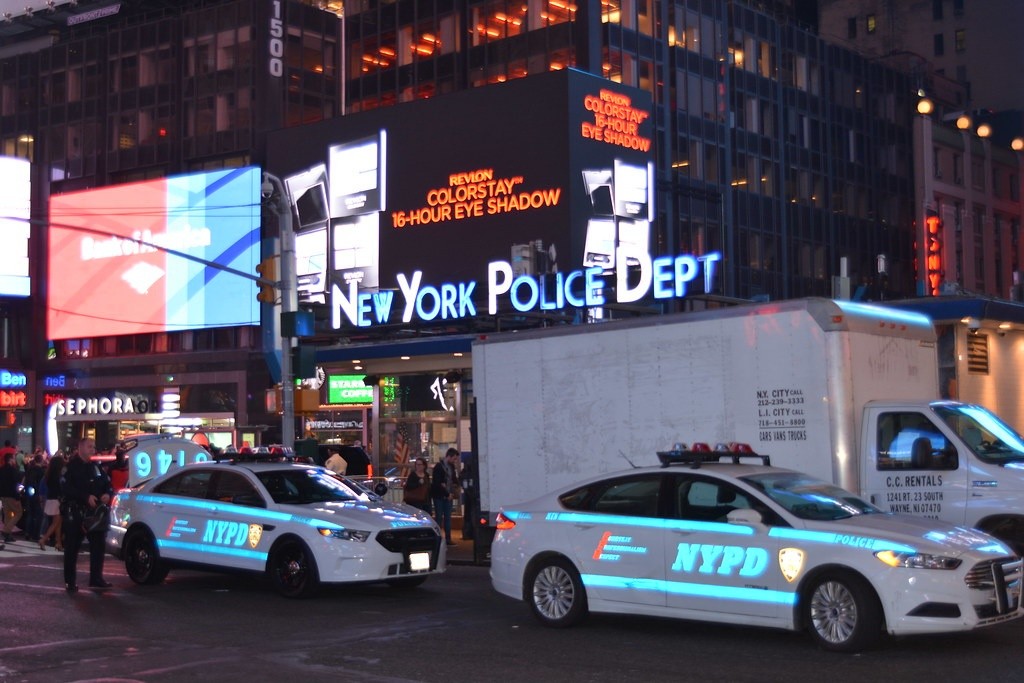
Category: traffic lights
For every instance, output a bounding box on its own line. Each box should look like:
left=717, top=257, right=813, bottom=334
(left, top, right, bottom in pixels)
left=255, top=259, right=280, bottom=304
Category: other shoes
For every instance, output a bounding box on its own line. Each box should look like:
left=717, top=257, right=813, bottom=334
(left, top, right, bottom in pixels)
left=4, top=538, right=16, bottom=542
left=64, top=582, right=79, bottom=592
left=89, top=575, right=112, bottom=588
left=446, top=540, right=458, bottom=548
left=12, top=526, right=22, bottom=532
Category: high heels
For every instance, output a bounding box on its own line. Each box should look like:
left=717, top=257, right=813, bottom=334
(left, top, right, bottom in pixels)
left=55, top=544, right=65, bottom=551
left=38, top=540, right=47, bottom=551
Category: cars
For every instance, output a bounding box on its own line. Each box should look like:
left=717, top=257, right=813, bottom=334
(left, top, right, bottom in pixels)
left=490, top=440, right=1024, bottom=647
left=316, top=443, right=372, bottom=480
left=103, top=434, right=446, bottom=598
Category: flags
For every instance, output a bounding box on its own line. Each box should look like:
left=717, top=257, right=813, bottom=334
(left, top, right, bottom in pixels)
left=393, top=418, right=412, bottom=486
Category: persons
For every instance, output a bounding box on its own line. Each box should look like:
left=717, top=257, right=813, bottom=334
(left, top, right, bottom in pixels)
left=432, top=448, right=462, bottom=547
left=403, top=456, right=434, bottom=518
left=0, top=439, right=157, bottom=552
left=323, top=446, right=349, bottom=479
left=63, top=438, right=113, bottom=594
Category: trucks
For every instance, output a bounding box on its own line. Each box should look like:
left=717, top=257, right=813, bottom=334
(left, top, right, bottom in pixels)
left=466, top=294, right=1024, bottom=576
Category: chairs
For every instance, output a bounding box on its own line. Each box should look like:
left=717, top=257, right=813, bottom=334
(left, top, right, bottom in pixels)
left=910, top=437, right=934, bottom=470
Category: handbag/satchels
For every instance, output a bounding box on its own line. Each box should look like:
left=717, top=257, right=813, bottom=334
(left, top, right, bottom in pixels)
left=403, top=484, right=428, bottom=503
left=81, top=501, right=110, bottom=540
